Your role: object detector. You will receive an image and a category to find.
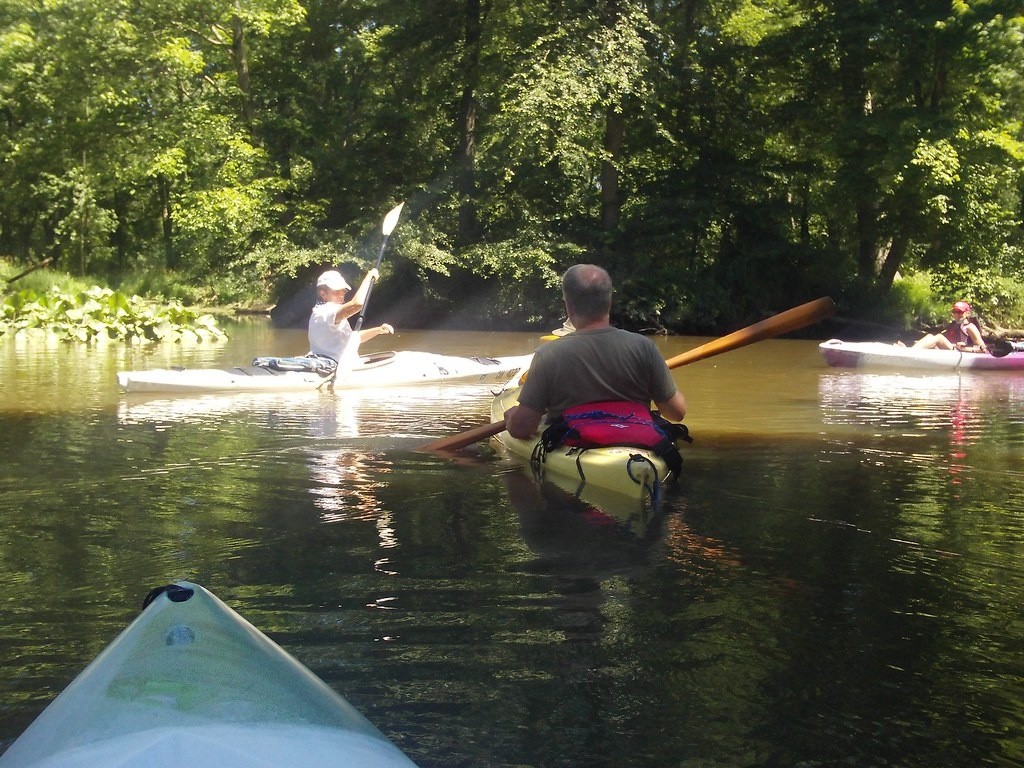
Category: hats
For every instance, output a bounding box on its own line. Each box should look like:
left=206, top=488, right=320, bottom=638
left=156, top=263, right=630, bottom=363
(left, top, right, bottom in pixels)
left=951, top=302, right=971, bottom=313
left=317, top=270, right=352, bottom=291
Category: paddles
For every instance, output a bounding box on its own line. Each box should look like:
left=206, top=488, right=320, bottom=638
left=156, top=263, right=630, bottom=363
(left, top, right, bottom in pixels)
left=423, top=296, right=840, bottom=453
left=904, top=337, right=1013, bottom=358
left=333, top=199, right=406, bottom=391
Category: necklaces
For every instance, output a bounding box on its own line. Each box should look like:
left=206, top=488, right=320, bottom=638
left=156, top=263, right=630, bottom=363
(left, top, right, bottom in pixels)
left=316, top=301, right=326, bottom=305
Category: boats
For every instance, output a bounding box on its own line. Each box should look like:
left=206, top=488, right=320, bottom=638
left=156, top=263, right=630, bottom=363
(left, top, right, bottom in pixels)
left=818, top=339, right=1024, bottom=369
left=116, top=349, right=534, bottom=392
left=491, top=440, right=661, bottom=539
left=118, top=382, right=511, bottom=420
left=490, top=361, right=676, bottom=499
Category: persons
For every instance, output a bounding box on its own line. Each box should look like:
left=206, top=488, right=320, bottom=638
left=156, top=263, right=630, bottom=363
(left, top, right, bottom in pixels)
left=308, top=270, right=394, bottom=364
left=503, top=263, right=687, bottom=440
left=897, top=301, right=989, bottom=353
